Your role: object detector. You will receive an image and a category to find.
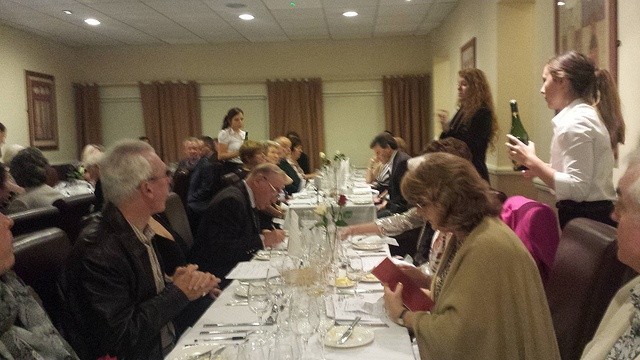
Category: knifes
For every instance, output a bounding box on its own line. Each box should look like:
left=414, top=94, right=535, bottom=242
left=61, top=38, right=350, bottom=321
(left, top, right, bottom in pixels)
left=200, top=330, right=250, bottom=334
left=338, top=288, right=388, bottom=293
left=203, top=322, right=265, bottom=327
left=337, top=316, right=361, bottom=343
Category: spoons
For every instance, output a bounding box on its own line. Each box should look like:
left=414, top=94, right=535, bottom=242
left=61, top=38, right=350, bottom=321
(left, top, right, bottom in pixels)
left=184, top=339, right=250, bottom=348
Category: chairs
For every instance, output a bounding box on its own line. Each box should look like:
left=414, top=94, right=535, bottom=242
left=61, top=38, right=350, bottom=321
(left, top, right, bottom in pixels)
left=540, top=218, right=622, bottom=359
left=5, top=204, right=59, bottom=237
left=77, top=213, right=105, bottom=229
left=507, top=195, right=540, bottom=254
left=9, top=226, right=70, bottom=302
left=492, top=183, right=502, bottom=202
left=57, top=191, right=100, bottom=232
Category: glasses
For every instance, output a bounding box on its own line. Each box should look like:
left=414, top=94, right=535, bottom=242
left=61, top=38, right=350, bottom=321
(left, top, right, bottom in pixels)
left=412, top=199, right=442, bottom=210
left=134, top=169, right=173, bottom=190
left=261, top=173, right=285, bottom=199
left=295, top=146, right=304, bottom=152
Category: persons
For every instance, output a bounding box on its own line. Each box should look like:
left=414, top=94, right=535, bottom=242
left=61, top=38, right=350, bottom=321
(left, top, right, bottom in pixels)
left=0, top=211, right=82, bottom=360
left=371, top=135, right=417, bottom=219
left=436, top=66, right=496, bottom=191
left=178, top=137, right=200, bottom=172
left=578, top=149, right=640, bottom=359
left=217, top=107, right=247, bottom=165
left=366, top=136, right=407, bottom=184
left=275, top=136, right=306, bottom=195
left=53, top=138, right=220, bottom=359
left=381, top=153, right=560, bottom=360
left=139, top=136, right=151, bottom=144
left=187, top=162, right=291, bottom=291
left=1, top=164, right=17, bottom=216
left=337, top=139, right=473, bottom=277
left=286, top=131, right=310, bottom=174
left=238, top=140, right=287, bottom=220
left=9, top=147, right=67, bottom=213
left=287, top=139, right=318, bottom=179
left=81, top=144, right=104, bottom=195
left=262, top=139, right=280, bottom=164
left=198, top=136, right=218, bottom=162
left=50, top=165, right=95, bottom=199
left=0, top=121, right=9, bottom=161
left=505, top=52, right=620, bottom=232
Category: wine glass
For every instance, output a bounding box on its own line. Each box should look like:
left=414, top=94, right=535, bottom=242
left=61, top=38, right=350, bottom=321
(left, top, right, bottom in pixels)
left=247, top=280, right=272, bottom=334
left=345, top=255, right=366, bottom=303
left=236, top=338, right=266, bottom=360
left=266, top=206, right=336, bottom=360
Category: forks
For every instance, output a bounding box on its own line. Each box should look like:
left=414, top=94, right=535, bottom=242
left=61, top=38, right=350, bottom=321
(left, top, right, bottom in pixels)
left=202, top=331, right=255, bottom=341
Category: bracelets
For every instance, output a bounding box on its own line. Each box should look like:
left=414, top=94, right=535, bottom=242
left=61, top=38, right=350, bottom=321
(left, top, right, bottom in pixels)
left=302, top=174, right=306, bottom=180
left=277, top=210, right=283, bottom=219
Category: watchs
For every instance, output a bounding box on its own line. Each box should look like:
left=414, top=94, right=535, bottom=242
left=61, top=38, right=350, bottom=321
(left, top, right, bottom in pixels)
left=396, top=308, right=408, bottom=326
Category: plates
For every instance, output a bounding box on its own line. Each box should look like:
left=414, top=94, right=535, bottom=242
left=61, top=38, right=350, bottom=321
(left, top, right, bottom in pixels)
left=324, top=325, right=375, bottom=349
left=352, top=169, right=370, bottom=194
left=351, top=197, right=372, bottom=205
left=233, top=283, right=249, bottom=296
left=351, top=234, right=385, bottom=250
